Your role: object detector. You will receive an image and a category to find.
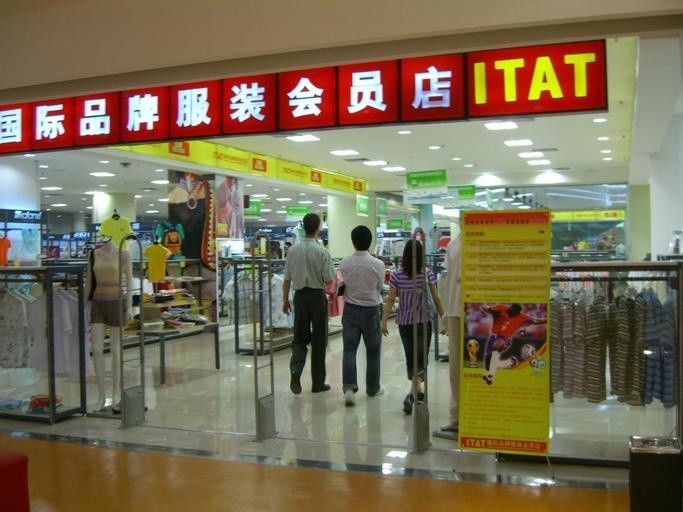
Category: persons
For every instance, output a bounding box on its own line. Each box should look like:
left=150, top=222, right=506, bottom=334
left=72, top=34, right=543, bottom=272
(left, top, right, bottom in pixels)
left=282, top=214, right=335, bottom=395
left=337, top=225, right=386, bottom=406
left=168, top=170, right=207, bottom=259
left=380, top=239, right=449, bottom=414
left=480, top=302, right=547, bottom=385
left=84, top=237, right=134, bottom=413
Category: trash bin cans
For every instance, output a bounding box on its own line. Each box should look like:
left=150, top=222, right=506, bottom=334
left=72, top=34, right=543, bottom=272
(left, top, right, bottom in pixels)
left=629, top=434, right=683, bottom=512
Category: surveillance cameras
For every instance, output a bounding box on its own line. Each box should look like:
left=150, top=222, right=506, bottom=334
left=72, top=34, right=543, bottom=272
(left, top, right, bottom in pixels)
left=120, top=163, right=130, bottom=167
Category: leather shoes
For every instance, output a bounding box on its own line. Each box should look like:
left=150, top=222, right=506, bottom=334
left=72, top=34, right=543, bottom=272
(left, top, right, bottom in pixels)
left=289, top=368, right=302, bottom=395
left=312, top=383, right=330, bottom=392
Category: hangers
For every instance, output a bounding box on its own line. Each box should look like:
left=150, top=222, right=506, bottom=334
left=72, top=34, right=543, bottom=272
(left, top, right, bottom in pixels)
left=552, top=273, right=672, bottom=295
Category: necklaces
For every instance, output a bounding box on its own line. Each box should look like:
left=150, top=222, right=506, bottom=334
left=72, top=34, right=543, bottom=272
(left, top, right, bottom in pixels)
left=178, top=176, right=201, bottom=209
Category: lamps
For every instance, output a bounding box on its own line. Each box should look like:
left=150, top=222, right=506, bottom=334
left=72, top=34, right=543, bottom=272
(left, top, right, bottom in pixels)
left=278, top=210, right=285, bottom=214
left=506, top=139, right=533, bottom=147
left=383, top=167, right=404, bottom=172
left=527, top=160, right=550, bottom=166
left=85, top=191, right=99, bottom=195
left=604, top=157, right=613, bottom=161
left=277, top=198, right=291, bottom=202
left=50, top=204, right=64, bottom=206
left=134, top=195, right=142, bottom=198
left=158, top=199, right=167, bottom=201
left=332, top=149, right=358, bottom=156
left=87, top=205, right=94, bottom=210
left=428, top=147, right=440, bottom=149
left=519, top=152, right=543, bottom=159
left=146, top=210, right=159, bottom=214
left=90, top=171, right=115, bottom=177
left=465, top=163, right=473, bottom=168
left=598, top=137, right=607, bottom=141
left=601, top=149, right=610, bottom=153
left=260, top=209, right=271, bottom=212
left=594, top=119, right=606, bottom=122
left=453, top=158, right=460, bottom=160
left=151, top=179, right=169, bottom=185
left=318, top=204, right=328, bottom=208
left=398, top=130, right=410, bottom=135
left=251, top=193, right=268, bottom=197
left=485, top=122, right=517, bottom=130
left=41, top=187, right=61, bottom=190
left=363, top=160, right=387, bottom=166
left=535, top=172, right=564, bottom=184
left=287, top=134, right=320, bottom=143
left=474, top=174, right=502, bottom=185
left=297, top=202, right=312, bottom=205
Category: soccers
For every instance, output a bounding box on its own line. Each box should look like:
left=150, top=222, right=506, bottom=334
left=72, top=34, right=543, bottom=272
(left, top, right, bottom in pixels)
left=521, top=344, right=536, bottom=360
left=538, top=360, right=546, bottom=368
left=531, top=358, right=538, bottom=366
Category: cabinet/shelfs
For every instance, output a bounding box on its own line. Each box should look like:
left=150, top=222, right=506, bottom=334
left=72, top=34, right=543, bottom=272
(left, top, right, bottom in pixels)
left=219, top=254, right=272, bottom=317
left=235, top=257, right=395, bottom=354
left=41, top=231, right=88, bottom=255
left=427, top=249, right=447, bottom=276
left=44, top=256, right=202, bottom=352
left=0, top=265, right=86, bottom=422
left=1, top=208, right=41, bottom=264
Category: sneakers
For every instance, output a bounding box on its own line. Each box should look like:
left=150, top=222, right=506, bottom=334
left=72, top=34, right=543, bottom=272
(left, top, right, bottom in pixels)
left=344, top=394, right=357, bottom=406
left=404, top=394, right=412, bottom=414
left=482, top=374, right=493, bottom=386
left=371, top=387, right=384, bottom=396
